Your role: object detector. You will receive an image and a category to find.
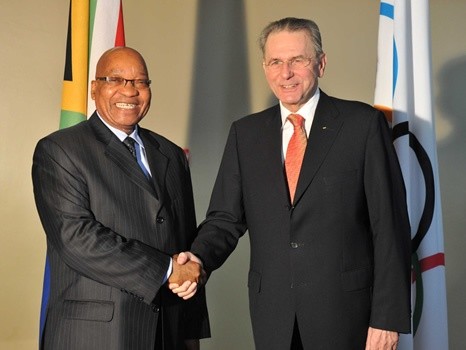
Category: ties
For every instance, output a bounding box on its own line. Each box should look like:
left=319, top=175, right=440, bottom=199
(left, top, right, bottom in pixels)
left=122, top=137, right=137, bottom=161
left=284, top=113, right=308, bottom=206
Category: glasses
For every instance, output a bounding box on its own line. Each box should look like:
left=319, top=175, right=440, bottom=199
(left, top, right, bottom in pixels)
left=96, top=77, right=151, bottom=89
left=265, top=56, right=318, bottom=69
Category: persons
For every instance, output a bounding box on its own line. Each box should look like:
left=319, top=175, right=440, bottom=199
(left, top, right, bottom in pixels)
left=30, top=46, right=212, bottom=350
left=167, top=16, right=415, bottom=350
